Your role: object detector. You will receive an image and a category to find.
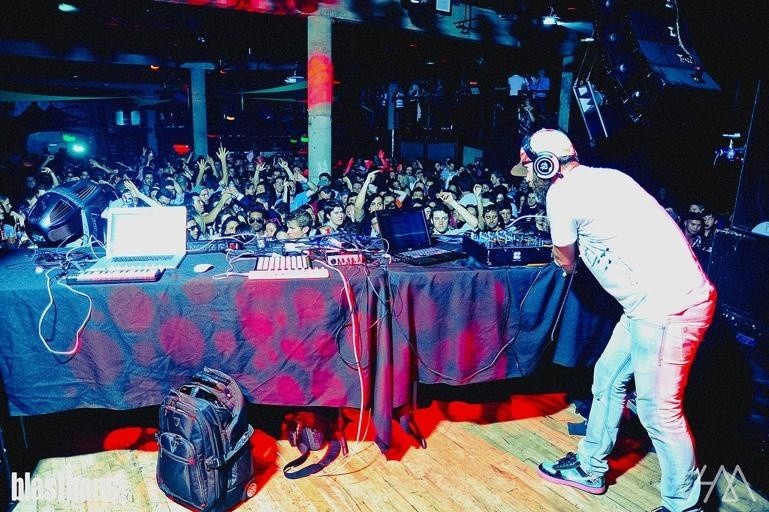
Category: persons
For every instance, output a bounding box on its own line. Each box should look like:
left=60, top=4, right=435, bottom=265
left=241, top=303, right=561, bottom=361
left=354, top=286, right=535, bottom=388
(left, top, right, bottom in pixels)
left=511, top=128, right=717, bottom=511
left=507, top=69, right=551, bottom=136
left=0, top=146, right=733, bottom=254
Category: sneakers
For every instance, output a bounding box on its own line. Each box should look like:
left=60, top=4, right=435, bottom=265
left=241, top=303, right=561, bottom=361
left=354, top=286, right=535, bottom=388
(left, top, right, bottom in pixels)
left=539, top=453, right=605, bottom=495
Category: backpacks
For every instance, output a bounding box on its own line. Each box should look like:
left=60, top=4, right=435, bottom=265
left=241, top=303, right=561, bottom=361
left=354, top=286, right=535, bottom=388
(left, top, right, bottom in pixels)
left=156, top=366, right=257, bottom=511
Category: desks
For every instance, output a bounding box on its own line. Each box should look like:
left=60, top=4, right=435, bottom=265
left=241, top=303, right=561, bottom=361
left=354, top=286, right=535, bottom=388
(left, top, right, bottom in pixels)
left=372, top=239, right=617, bottom=448
left=1, top=237, right=395, bottom=512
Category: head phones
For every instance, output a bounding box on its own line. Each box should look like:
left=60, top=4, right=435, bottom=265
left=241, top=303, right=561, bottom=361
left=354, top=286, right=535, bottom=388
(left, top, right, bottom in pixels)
left=522, top=134, right=579, bottom=180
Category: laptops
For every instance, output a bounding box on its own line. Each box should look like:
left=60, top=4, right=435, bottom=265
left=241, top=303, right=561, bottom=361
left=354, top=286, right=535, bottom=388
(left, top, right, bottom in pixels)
left=375, top=207, right=469, bottom=266
left=85, top=207, right=187, bottom=270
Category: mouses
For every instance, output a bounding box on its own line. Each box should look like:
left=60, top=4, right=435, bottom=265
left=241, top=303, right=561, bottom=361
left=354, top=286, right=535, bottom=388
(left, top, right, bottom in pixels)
left=194, top=264, right=214, bottom=272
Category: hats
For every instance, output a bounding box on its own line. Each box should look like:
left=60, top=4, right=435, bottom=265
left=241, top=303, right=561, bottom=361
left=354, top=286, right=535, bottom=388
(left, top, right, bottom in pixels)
left=520, top=128, right=580, bottom=163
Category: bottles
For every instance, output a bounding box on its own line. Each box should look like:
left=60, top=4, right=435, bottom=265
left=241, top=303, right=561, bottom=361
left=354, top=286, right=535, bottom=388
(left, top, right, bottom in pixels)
left=15, top=216, right=23, bottom=241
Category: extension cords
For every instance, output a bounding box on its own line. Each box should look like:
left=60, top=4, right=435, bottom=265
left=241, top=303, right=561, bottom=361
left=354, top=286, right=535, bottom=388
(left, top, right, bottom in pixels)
left=187, top=240, right=228, bottom=253
left=327, top=255, right=363, bottom=266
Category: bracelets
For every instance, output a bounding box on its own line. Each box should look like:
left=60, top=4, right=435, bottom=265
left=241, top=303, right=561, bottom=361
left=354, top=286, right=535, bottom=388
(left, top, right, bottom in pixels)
left=305, top=178, right=309, bottom=185
